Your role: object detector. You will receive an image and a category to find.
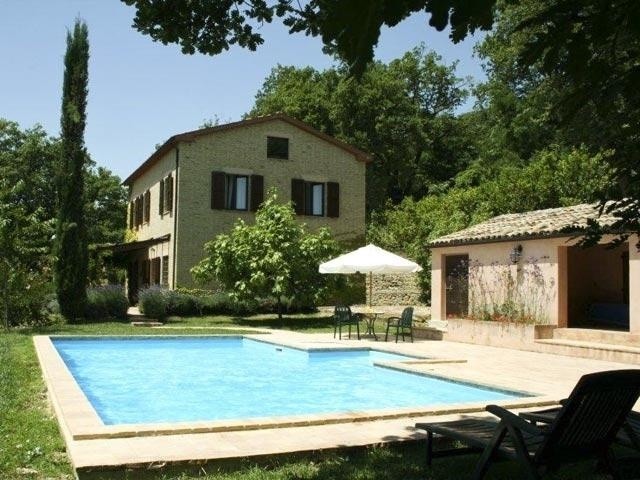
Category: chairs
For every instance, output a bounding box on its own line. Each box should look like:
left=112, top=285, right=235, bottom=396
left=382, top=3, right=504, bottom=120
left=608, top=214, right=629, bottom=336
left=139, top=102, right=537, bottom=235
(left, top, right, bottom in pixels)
left=334, top=304, right=359, bottom=340
left=416, top=370, right=640, bottom=480
left=519, top=407, right=640, bottom=453
left=385, top=308, right=413, bottom=343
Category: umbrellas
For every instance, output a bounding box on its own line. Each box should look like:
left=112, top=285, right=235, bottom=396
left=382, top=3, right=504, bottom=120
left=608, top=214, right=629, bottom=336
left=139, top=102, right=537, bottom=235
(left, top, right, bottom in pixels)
left=319, top=242, right=424, bottom=309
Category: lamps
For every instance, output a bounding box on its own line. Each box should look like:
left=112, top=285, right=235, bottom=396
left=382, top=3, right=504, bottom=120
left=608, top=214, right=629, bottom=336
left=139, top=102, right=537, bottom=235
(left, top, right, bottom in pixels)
left=510, top=245, right=522, bottom=263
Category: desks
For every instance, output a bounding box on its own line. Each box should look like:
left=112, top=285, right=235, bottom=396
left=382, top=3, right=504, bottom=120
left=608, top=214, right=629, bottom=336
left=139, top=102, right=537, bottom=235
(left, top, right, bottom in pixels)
left=352, top=310, right=385, bottom=341
